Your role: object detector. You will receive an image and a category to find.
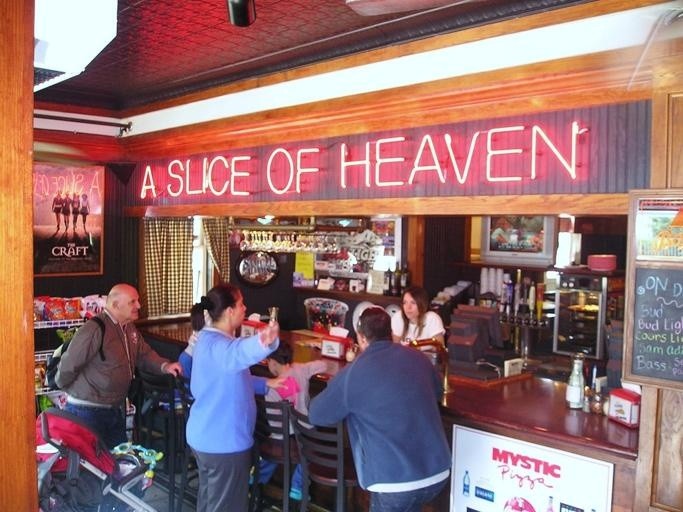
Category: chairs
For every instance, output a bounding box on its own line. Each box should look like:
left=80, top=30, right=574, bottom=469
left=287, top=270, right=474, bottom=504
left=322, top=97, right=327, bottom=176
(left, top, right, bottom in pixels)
left=128, top=366, right=363, bottom=512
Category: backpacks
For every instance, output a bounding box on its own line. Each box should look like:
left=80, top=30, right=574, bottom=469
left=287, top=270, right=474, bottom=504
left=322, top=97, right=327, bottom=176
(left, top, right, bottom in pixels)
left=42, top=315, right=106, bottom=394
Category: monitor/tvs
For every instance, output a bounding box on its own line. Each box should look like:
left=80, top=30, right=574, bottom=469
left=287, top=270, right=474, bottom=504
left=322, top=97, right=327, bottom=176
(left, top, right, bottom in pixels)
left=454, top=303, right=504, bottom=350
left=480, top=215, right=558, bottom=268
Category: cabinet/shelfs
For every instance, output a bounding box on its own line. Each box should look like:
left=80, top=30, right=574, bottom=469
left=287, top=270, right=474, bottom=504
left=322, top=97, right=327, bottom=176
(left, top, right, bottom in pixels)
left=33, top=315, right=133, bottom=443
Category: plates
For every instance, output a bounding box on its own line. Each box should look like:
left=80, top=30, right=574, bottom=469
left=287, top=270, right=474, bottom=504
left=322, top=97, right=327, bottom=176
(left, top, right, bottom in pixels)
left=588, top=254, right=617, bottom=271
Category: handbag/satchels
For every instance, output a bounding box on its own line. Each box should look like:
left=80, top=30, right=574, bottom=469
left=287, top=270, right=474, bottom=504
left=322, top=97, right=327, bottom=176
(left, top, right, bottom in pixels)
left=37, top=440, right=101, bottom=511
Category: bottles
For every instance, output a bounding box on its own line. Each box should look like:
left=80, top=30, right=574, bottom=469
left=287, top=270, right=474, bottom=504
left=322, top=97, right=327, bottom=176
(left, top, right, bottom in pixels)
left=383, top=261, right=410, bottom=298
left=346, top=349, right=355, bottom=363
left=564, top=353, right=603, bottom=415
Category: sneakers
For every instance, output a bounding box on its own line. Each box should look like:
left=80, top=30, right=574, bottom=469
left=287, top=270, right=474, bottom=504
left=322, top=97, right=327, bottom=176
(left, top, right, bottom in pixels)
left=289, top=488, right=313, bottom=503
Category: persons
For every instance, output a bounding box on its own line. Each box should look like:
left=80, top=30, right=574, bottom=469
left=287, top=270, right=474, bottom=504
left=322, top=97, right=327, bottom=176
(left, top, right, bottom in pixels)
left=388, top=285, right=447, bottom=354
left=308, top=305, right=453, bottom=511
left=182, top=286, right=280, bottom=511
left=51, top=189, right=90, bottom=232
left=247, top=341, right=328, bottom=504
left=174, top=302, right=290, bottom=401
left=54, top=282, right=185, bottom=453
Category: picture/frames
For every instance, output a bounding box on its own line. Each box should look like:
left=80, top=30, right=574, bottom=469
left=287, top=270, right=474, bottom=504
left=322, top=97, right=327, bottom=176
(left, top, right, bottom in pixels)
left=33, top=164, right=106, bottom=278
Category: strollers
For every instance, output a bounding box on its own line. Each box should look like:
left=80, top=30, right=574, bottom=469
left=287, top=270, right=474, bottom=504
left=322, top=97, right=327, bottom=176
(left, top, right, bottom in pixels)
left=37, top=408, right=164, bottom=512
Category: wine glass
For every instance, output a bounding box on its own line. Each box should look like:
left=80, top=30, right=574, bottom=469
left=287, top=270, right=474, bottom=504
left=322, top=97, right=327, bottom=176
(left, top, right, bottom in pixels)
left=240, top=229, right=340, bottom=254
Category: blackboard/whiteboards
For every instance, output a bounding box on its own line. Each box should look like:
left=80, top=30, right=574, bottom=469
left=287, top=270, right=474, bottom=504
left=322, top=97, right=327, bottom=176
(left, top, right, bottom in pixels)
left=622, top=261, right=683, bottom=391
